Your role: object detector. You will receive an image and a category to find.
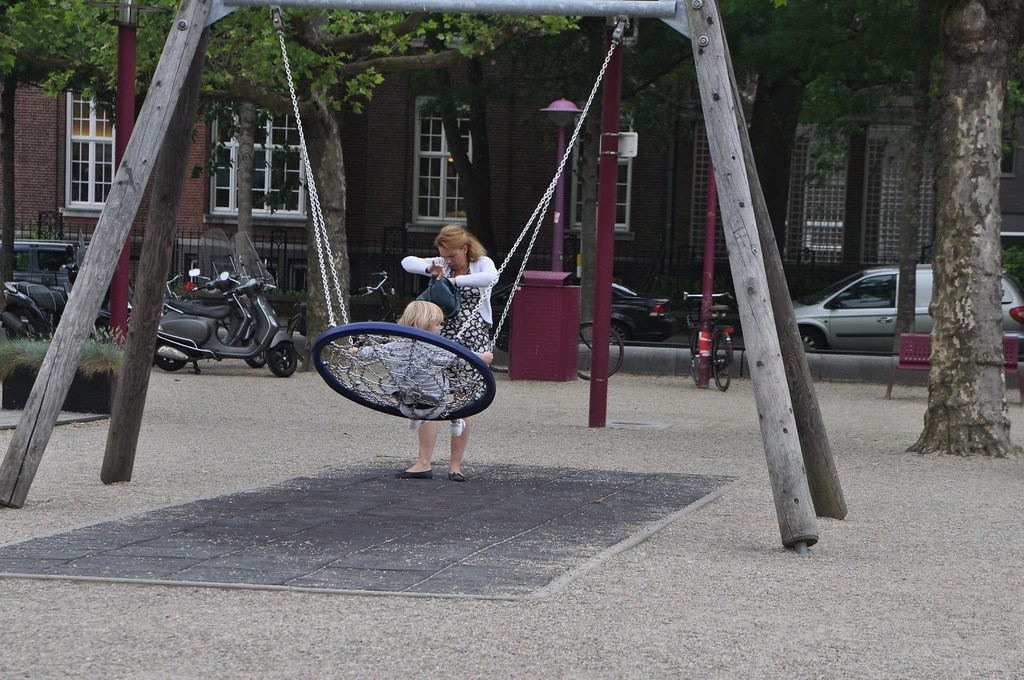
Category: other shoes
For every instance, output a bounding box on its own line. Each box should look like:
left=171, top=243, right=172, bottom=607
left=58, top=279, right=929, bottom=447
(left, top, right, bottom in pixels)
left=394, top=468, right=433, bottom=479
left=448, top=470, right=466, bottom=482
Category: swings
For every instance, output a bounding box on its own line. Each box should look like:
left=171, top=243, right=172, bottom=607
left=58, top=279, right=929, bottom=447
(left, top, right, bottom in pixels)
left=272, top=19, right=627, bottom=422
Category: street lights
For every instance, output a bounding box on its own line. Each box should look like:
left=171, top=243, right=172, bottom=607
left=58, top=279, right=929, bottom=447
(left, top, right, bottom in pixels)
left=537, top=95, right=582, bottom=273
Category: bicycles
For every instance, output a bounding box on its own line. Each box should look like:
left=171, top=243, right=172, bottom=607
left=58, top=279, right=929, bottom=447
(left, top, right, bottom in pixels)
left=288, top=273, right=401, bottom=363
left=682, top=291, right=736, bottom=392
left=471, top=295, right=624, bottom=380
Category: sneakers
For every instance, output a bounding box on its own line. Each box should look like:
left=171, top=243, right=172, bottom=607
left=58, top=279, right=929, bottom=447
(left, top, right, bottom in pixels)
left=449, top=419, right=465, bottom=436
left=408, top=419, right=423, bottom=430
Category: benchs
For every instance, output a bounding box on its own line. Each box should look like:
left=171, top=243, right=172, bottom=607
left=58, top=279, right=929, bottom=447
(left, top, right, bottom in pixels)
left=886, top=333, right=1024, bottom=406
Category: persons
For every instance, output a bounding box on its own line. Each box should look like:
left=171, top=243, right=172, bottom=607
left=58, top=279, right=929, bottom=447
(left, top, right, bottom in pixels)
left=395, top=225, right=498, bottom=481
left=344, top=301, right=493, bottom=436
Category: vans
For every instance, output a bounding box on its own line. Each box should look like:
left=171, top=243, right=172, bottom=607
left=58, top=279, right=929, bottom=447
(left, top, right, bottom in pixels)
left=0, top=235, right=80, bottom=293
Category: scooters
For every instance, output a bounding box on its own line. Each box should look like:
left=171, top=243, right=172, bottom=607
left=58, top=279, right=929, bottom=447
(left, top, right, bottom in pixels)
left=0, top=246, right=299, bottom=383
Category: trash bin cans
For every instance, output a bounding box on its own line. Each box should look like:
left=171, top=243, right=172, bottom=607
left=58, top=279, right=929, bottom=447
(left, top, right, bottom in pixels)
left=508, top=270, right=582, bottom=382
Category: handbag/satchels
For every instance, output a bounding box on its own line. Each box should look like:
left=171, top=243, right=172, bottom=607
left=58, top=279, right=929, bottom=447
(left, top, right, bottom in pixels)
left=415, top=267, right=461, bottom=318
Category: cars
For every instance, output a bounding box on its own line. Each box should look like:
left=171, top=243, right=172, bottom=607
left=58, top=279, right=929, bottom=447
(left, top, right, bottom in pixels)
left=483, top=270, right=676, bottom=349
left=789, top=265, right=1024, bottom=361
